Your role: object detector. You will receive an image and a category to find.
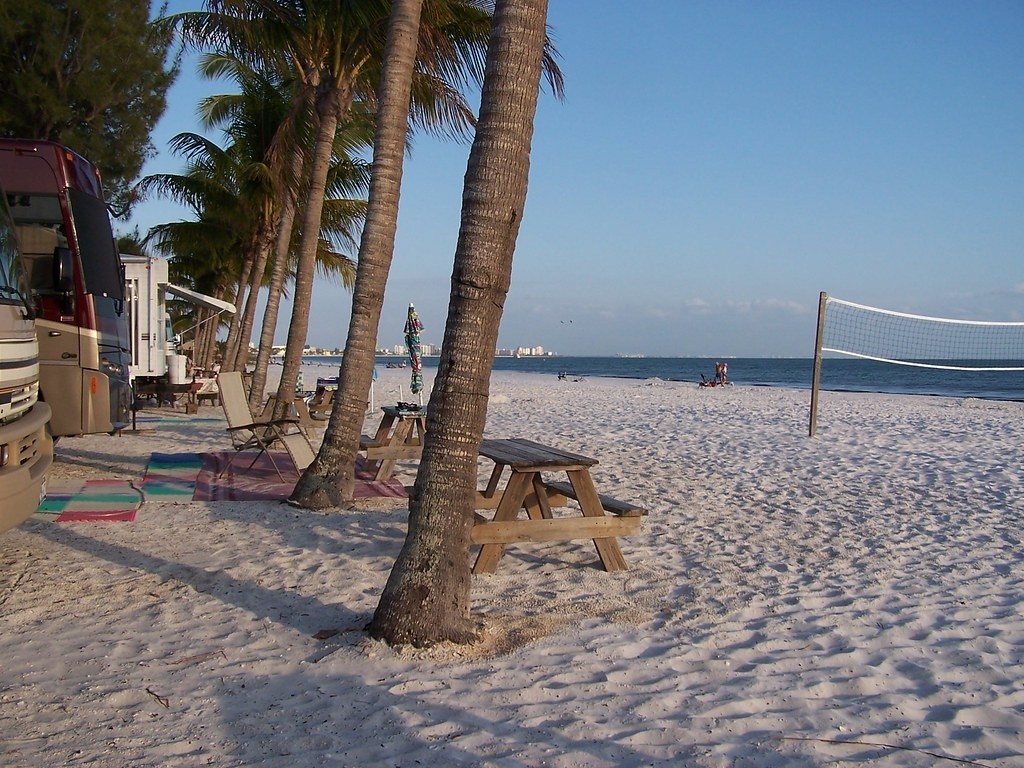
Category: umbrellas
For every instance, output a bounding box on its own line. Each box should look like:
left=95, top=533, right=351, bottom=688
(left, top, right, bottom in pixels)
left=403, top=303, right=424, bottom=406
left=371, top=368, right=378, bottom=413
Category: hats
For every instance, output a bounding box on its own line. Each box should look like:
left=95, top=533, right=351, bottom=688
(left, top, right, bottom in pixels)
left=724, top=363, right=728, bottom=366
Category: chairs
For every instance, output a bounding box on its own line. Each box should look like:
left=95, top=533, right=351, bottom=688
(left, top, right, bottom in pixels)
left=215, top=370, right=317, bottom=484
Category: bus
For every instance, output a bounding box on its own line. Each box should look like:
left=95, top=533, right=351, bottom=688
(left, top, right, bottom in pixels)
left=0, top=179, right=55, bottom=534
left=0, top=138, right=132, bottom=447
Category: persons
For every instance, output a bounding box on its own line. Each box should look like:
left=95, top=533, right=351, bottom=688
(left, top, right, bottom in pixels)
left=714, top=362, right=728, bottom=387
left=386, top=358, right=406, bottom=368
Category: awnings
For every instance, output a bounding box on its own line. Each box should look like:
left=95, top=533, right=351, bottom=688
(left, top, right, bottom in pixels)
left=160, top=282, right=236, bottom=342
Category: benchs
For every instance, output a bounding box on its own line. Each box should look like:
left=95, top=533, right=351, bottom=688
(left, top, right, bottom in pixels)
left=402, top=486, right=486, bottom=527
left=359, top=434, right=383, bottom=449
left=311, top=412, right=331, bottom=421
left=545, top=481, right=648, bottom=517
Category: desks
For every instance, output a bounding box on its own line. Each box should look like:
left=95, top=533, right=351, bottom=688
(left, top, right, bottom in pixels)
left=359, top=403, right=428, bottom=478
left=307, top=384, right=338, bottom=416
left=260, top=391, right=313, bottom=425
left=466, top=437, right=648, bottom=573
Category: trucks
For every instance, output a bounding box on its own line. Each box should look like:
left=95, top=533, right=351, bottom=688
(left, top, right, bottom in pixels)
left=119, top=252, right=236, bottom=410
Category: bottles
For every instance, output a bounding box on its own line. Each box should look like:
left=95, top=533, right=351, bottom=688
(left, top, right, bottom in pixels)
left=290, top=402, right=297, bottom=417
